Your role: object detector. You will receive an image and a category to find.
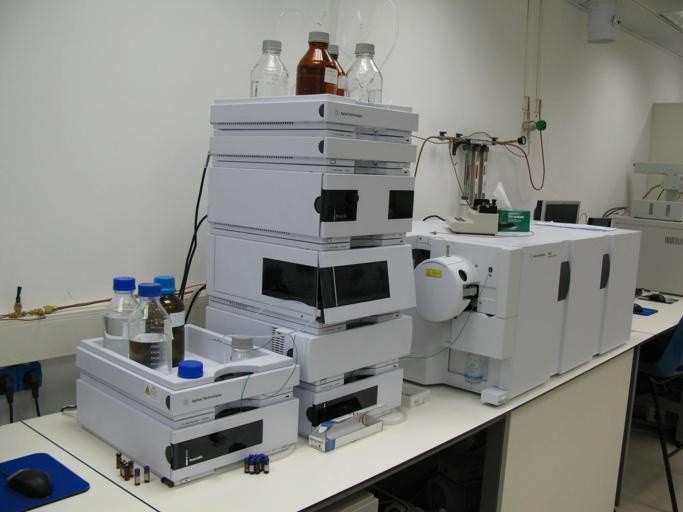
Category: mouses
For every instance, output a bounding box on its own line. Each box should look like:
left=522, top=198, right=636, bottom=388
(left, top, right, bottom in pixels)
left=632, top=303, right=643, bottom=313
left=648, top=292, right=666, bottom=303
left=7, top=467, right=53, bottom=498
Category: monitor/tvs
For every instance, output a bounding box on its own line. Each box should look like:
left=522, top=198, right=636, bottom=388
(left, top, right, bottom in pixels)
left=587, top=216, right=612, bottom=226
left=532, top=200, right=581, bottom=223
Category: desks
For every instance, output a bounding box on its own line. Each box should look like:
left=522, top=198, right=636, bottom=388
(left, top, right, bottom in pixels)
left=0, top=290, right=683, bottom=512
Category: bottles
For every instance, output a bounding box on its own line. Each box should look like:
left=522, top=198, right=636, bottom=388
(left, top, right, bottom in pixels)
left=294, top=30, right=339, bottom=96
left=151, top=274, right=187, bottom=369
left=103, top=277, right=140, bottom=360
left=128, top=282, right=173, bottom=380
left=345, top=42, right=382, bottom=105
left=250, top=40, right=289, bottom=98
left=323, top=42, right=346, bottom=96
